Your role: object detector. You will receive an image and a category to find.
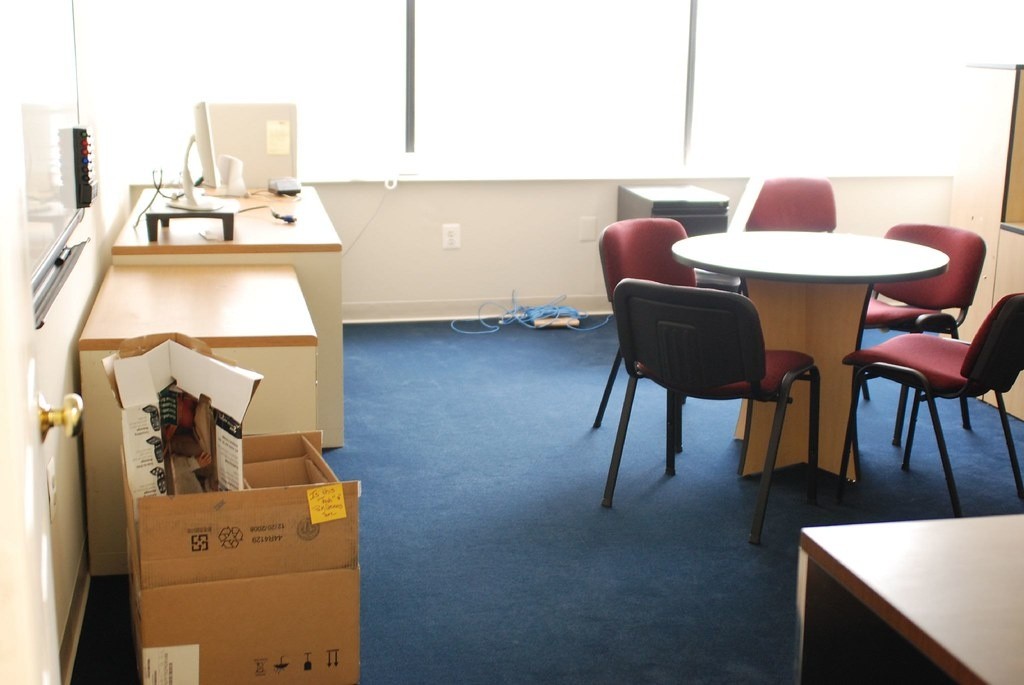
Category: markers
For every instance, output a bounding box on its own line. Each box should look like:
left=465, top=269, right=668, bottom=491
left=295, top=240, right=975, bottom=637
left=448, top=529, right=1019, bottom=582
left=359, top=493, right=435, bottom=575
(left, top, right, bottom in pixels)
left=54, top=248, right=70, bottom=266
left=81, top=128, right=96, bottom=183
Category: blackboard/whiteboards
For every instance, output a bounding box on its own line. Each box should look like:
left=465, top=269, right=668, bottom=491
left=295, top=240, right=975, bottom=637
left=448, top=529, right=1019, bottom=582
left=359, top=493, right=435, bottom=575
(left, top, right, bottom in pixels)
left=13, top=0, right=98, bottom=330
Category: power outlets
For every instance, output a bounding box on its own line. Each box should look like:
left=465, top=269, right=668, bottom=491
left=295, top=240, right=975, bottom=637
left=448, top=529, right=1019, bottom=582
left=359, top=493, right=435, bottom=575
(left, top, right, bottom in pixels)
left=47, top=458, right=59, bottom=521
left=442, top=223, right=461, bottom=250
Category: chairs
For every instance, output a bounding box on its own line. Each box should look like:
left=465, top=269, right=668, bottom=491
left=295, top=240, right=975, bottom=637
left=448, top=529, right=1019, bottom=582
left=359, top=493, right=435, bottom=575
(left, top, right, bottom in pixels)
left=745, top=177, right=836, bottom=234
left=601, top=276, right=821, bottom=547
left=860, top=226, right=987, bottom=445
left=838, top=292, right=1024, bottom=516
left=591, top=218, right=696, bottom=453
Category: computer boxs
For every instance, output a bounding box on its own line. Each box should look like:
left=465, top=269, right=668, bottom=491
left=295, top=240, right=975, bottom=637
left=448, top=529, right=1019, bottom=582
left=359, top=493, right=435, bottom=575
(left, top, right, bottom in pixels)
left=207, top=102, right=297, bottom=189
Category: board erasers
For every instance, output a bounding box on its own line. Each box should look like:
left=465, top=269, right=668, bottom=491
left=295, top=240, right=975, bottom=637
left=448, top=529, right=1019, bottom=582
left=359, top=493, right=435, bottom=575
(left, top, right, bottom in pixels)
left=79, top=181, right=98, bottom=203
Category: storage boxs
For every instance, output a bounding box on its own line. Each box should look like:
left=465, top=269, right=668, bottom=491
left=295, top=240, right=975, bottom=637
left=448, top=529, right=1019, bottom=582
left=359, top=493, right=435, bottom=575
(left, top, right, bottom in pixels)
left=118, top=432, right=361, bottom=685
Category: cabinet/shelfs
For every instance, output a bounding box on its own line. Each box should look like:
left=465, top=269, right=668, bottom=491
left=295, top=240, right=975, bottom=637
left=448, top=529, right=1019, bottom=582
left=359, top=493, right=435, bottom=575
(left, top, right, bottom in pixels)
left=617, top=186, right=729, bottom=238
left=79, top=186, right=345, bottom=579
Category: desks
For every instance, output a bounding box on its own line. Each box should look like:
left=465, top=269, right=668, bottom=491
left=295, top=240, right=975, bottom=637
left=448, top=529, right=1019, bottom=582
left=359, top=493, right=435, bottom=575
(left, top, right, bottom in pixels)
left=670, top=232, right=951, bottom=489
left=792, top=512, right=1024, bottom=685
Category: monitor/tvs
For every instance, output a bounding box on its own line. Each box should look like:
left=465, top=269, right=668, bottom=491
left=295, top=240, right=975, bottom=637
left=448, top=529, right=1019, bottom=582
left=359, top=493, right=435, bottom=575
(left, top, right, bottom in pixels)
left=165, top=101, right=223, bottom=211
